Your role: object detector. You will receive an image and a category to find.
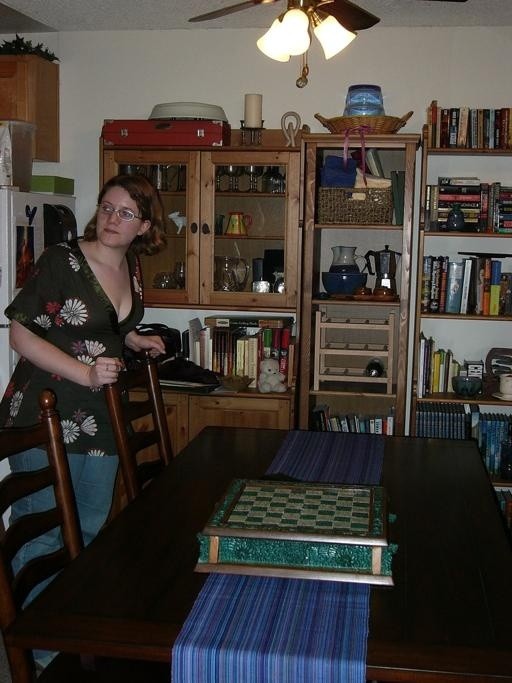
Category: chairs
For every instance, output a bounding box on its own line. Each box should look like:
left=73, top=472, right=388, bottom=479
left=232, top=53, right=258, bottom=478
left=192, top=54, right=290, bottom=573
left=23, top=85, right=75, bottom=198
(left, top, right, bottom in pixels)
left=0, top=389, right=172, bottom=683
left=95, top=346, right=177, bottom=508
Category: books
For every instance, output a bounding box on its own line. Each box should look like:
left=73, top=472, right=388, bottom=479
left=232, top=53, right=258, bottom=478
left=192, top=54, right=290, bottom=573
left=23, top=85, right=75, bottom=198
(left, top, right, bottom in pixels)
left=350, top=148, right=405, bottom=227
left=181, top=314, right=295, bottom=390
left=312, top=405, right=394, bottom=436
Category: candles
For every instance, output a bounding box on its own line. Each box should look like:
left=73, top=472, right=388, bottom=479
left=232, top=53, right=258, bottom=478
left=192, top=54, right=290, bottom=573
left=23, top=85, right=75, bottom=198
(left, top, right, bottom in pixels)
left=241, top=90, right=265, bottom=129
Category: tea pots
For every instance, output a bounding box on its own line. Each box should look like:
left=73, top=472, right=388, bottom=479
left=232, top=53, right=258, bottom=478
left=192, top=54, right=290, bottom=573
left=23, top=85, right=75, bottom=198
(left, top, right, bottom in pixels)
left=224, top=211, right=253, bottom=237
left=328, top=245, right=367, bottom=274
left=119, top=163, right=186, bottom=191
left=213, top=254, right=250, bottom=293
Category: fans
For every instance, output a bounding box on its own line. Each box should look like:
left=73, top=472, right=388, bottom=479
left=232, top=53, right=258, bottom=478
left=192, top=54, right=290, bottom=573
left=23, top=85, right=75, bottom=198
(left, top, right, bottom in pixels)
left=178, top=0, right=469, bottom=43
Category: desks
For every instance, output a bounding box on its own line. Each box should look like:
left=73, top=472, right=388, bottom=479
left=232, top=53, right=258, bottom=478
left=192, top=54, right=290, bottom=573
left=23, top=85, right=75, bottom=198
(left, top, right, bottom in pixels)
left=6, top=419, right=511, bottom=683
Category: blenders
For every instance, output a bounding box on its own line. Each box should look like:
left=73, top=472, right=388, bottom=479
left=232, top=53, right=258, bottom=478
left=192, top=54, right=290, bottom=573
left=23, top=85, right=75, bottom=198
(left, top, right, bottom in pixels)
left=364, top=244, right=401, bottom=295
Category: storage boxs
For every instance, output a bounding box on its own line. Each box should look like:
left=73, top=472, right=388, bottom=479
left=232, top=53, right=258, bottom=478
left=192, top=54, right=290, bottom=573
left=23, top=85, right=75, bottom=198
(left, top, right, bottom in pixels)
left=27, top=173, right=75, bottom=195
left=0, top=120, right=38, bottom=192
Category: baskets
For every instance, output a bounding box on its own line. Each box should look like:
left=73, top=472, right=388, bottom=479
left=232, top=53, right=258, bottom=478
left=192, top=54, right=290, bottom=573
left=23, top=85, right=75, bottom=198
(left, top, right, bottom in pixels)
left=316, top=185, right=394, bottom=225
left=314, top=110, right=415, bottom=134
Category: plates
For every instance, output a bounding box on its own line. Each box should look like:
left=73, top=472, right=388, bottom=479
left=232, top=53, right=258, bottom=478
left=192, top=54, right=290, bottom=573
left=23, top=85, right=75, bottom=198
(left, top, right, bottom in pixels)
left=491, top=391, right=512, bottom=402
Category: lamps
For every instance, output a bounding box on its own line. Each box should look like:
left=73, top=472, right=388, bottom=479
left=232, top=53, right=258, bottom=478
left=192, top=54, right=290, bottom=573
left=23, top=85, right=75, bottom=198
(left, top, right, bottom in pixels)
left=251, top=0, right=358, bottom=89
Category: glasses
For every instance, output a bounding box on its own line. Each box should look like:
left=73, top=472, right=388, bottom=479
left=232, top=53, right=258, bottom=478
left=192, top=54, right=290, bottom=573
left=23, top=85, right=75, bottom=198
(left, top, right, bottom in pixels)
left=96, top=204, right=145, bottom=222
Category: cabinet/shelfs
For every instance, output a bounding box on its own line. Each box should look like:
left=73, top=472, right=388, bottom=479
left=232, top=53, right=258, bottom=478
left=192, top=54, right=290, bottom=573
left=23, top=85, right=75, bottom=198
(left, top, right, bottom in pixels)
left=103, top=383, right=300, bottom=533
left=95, top=120, right=306, bottom=314
left=400, top=121, right=511, bottom=494
left=295, top=123, right=420, bottom=437
left=1, top=52, right=61, bottom=164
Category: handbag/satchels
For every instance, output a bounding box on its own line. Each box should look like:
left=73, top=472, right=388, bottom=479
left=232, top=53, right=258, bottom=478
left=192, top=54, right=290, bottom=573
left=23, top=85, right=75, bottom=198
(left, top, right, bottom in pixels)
left=124, top=322, right=183, bottom=388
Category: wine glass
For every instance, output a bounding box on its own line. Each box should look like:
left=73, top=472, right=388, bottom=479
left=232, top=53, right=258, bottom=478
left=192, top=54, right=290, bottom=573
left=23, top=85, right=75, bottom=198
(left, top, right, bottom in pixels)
left=174, top=262, right=186, bottom=290
left=214, top=162, right=265, bottom=194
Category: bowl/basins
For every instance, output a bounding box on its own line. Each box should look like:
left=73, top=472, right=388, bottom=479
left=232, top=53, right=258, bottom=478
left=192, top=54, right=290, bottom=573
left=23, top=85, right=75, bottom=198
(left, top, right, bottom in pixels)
left=148, top=101, right=229, bottom=123
left=216, top=374, right=255, bottom=392
left=320, top=270, right=368, bottom=296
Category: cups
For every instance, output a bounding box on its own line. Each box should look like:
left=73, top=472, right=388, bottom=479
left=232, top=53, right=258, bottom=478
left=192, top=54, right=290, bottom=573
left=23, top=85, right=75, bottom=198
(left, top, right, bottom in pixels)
left=451, top=375, right=486, bottom=399
left=353, top=286, right=397, bottom=301
left=252, top=280, right=271, bottom=292
left=215, top=213, right=225, bottom=234
left=251, top=256, right=265, bottom=280
left=499, top=373, right=511, bottom=398
left=391, top=169, right=404, bottom=225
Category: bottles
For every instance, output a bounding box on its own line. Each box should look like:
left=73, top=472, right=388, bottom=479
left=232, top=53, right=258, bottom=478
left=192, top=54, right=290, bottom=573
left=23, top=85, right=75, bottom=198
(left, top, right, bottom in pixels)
left=263, top=166, right=284, bottom=194
left=273, top=272, right=285, bottom=293
left=445, top=203, right=466, bottom=232
left=153, top=272, right=176, bottom=290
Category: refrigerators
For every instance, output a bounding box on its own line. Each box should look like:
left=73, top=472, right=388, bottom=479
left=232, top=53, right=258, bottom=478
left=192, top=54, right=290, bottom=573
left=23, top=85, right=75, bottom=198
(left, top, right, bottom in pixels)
left=1, top=185, right=81, bottom=402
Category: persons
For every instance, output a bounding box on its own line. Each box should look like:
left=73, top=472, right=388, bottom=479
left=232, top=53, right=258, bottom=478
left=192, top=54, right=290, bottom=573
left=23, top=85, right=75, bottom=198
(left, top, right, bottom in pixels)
left=0, top=174, right=166, bottom=667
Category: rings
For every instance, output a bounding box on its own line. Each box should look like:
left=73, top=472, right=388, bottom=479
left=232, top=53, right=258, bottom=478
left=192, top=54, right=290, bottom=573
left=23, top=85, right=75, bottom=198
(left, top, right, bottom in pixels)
left=106, top=363, right=109, bottom=372
